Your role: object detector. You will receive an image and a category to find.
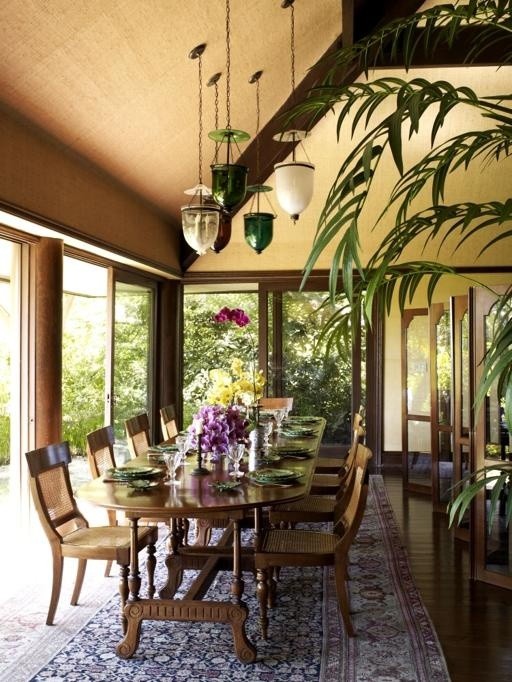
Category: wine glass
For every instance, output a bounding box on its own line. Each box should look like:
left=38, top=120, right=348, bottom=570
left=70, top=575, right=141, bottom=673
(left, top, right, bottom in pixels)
left=164, top=483, right=183, bottom=508
left=273, top=409, right=285, bottom=431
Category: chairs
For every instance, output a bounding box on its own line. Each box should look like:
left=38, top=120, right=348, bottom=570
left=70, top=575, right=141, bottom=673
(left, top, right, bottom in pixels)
left=259, top=397, right=294, bottom=418
left=24, top=440, right=161, bottom=636
left=159, top=403, right=179, bottom=441
left=255, top=443, right=372, bottom=640
left=124, top=413, right=181, bottom=551
left=269, top=405, right=369, bottom=606
left=85, top=424, right=188, bottom=579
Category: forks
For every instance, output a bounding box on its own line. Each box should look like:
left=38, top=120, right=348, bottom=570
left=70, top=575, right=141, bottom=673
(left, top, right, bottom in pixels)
left=252, top=480, right=294, bottom=488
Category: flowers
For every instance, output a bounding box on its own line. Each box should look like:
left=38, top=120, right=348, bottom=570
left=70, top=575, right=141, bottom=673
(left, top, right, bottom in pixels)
left=186, top=405, right=246, bottom=458
left=206, top=306, right=266, bottom=405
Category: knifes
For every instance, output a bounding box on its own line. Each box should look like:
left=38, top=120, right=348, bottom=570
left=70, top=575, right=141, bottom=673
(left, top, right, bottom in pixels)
left=103, top=478, right=131, bottom=483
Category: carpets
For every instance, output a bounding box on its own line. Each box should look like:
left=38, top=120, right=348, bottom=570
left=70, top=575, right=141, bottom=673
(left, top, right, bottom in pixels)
left=0, top=473, right=452, bottom=682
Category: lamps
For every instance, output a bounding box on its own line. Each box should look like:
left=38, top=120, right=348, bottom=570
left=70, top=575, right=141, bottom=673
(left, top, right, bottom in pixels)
left=180, top=0, right=315, bottom=257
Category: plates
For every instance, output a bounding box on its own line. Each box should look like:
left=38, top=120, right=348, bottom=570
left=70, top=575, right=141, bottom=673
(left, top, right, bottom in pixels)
left=107, top=429, right=189, bottom=489
left=244, top=415, right=323, bottom=482
left=207, top=478, right=242, bottom=489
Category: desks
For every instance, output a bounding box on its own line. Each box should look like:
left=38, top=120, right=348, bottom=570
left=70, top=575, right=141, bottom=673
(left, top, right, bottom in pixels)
left=73, top=413, right=326, bottom=664
